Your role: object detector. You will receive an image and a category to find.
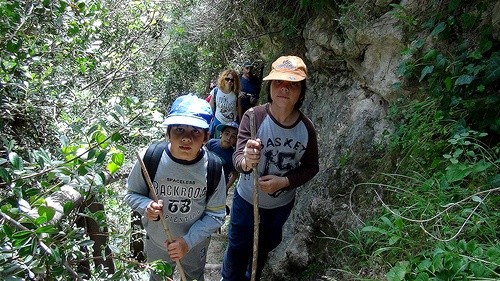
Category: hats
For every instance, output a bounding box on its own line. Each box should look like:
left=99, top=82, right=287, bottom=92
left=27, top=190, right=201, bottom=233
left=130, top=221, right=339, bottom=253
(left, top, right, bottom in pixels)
left=216, top=121, right=239, bottom=130
left=162, top=95, right=213, bottom=128
left=242, top=62, right=253, bottom=68
left=263, top=56, right=307, bottom=82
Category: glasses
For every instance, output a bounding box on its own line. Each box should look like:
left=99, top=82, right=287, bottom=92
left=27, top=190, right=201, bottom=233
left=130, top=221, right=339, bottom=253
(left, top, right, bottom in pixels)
left=225, top=77, right=234, bottom=82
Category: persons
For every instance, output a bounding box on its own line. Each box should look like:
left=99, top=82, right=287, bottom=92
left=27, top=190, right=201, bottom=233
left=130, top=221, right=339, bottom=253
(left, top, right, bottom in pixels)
left=234, top=59, right=261, bottom=123
left=205, top=122, right=239, bottom=195
left=222, top=56, right=319, bottom=281
left=124, top=95, right=227, bottom=281
left=209, top=69, right=241, bottom=138
